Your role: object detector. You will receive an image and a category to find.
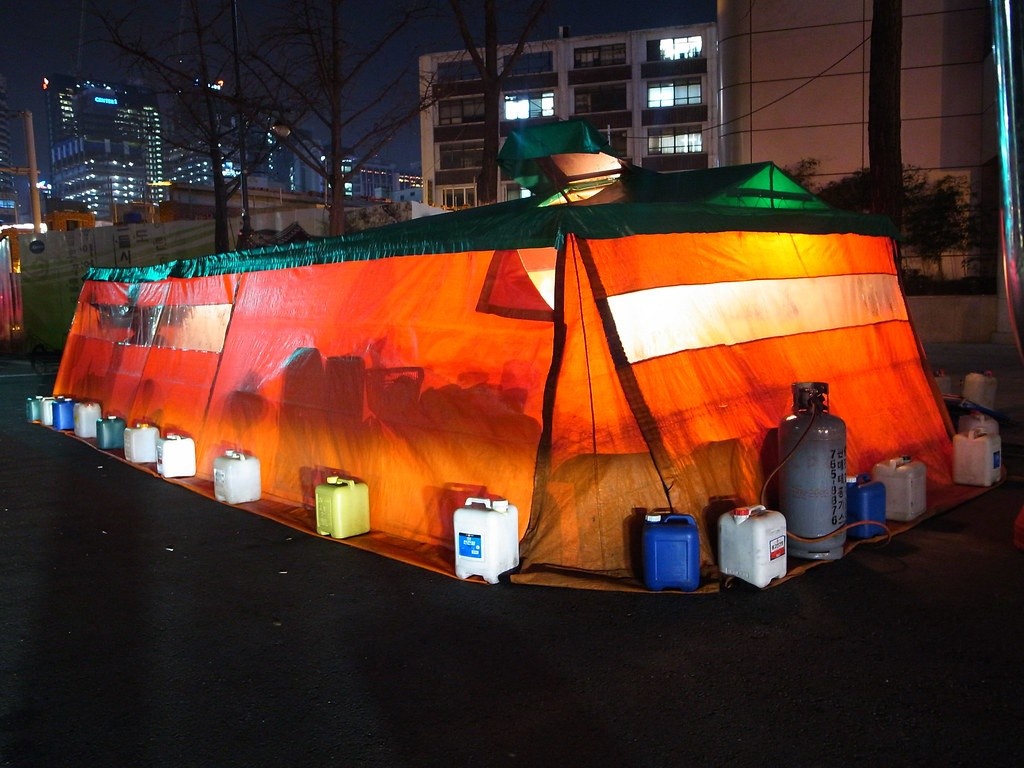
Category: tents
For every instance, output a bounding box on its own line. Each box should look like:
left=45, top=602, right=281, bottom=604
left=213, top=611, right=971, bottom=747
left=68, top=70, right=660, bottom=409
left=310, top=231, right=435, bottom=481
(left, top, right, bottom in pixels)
left=27, top=118, right=1007, bottom=594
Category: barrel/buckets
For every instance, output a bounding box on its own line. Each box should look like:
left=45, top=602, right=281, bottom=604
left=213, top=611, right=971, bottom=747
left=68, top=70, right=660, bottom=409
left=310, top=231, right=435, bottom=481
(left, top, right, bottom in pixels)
left=52, top=398, right=78, bottom=430
left=846, top=473, right=887, bottom=538
left=641, top=513, right=700, bottom=592
left=73, top=401, right=102, bottom=438
left=213, top=449, right=261, bottom=505
left=717, top=503, right=786, bottom=588
left=963, top=371, right=998, bottom=410
left=315, top=475, right=371, bottom=539
left=40, top=397, right=56, bottom=425
left=25, top=395, right=43, bottom=421
left=871, top=455, right=927, bottom=521
left=123, top=423, right=160, bottom=463
left=952, top=412, right=1002, bottom=487
left=933, top=368, right=952, bottom=394
left=452, top=496, right=519, bottom=584
left=155, top=432, right=196, bottom=478
left=95, top=415, right=126, bottom=450
